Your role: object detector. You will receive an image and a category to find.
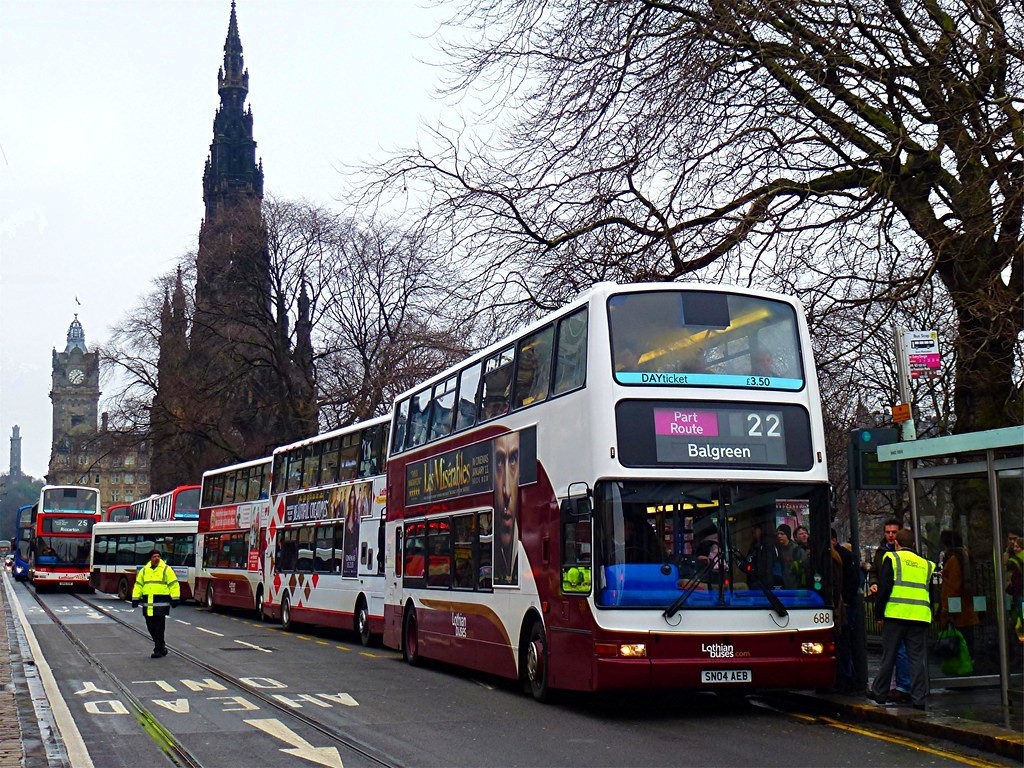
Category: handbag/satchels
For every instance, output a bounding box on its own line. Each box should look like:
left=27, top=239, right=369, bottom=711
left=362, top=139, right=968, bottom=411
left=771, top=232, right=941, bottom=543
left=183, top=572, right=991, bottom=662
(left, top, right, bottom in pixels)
left=935, top=621, right=973, bottom=675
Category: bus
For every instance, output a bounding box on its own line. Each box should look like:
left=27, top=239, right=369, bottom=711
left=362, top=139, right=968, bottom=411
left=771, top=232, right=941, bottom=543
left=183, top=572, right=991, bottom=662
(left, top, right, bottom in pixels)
left=0, top=541, right=11, bottom=557
left=11, top=504, right=34, bottom=582
left=129, top=485, right=201, bottom=521
left=88, top=520, right=199, bottom=605
left=194, top=455, right=274, bottom=623
left=29, top=485, right=101, bottom=594
left=101, top=504, right=130, bottom=522
left=262, top=412, right=394, bottom=648
left=384, top=281, right=840, bottom=704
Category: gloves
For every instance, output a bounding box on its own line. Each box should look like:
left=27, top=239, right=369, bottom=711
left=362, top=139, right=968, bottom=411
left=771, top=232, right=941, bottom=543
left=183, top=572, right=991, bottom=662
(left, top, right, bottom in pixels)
left=172, top=599, right=180, bottom=608
left=132, top=600, right=140, bottom=608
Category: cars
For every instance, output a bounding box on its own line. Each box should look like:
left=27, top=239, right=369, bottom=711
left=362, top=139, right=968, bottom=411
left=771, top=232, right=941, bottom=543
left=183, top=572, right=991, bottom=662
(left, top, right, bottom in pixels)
left=4, top=555, right=15, bottom=561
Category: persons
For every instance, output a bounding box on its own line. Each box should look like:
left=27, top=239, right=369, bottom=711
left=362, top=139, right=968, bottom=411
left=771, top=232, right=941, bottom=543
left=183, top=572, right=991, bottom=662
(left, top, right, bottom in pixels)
left=692, top=518, right=729, bottom=584
left=1003, top=528, right=1024, bottom=675
left=639, top=517, right=670, bottom=563
left=869, top=518, right=910, bottom=699
left=434, top=543, right=442, bottom=554
left=940, top=530, right=980, bottom=689
left=333, top=484, right=370, bottom=576
left=776, top=523, right=868, bottom=694
left=746, top=520, right=784, bottom=590
left=132, top=549, right=181, bottom=658
left=493, top=431, right=520, bottom=585
left=866, top=528, right=936, bottom=710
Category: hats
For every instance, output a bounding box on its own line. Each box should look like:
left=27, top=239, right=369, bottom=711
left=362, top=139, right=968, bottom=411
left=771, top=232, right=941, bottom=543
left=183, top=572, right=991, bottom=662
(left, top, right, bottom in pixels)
left=777, top=524, right=791, bottom=539
left=149, top=549, right=161, bottom=558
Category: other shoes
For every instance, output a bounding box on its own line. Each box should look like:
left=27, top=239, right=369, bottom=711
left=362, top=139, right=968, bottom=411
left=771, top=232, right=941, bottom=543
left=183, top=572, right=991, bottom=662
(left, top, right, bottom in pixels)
left=866, top=690, right=886, bottom=704
left=887, top=689, right=911, bottom=700
left=151, top=652, right=164, bottom=658
left=913, top=702, right=925, bottom=711
